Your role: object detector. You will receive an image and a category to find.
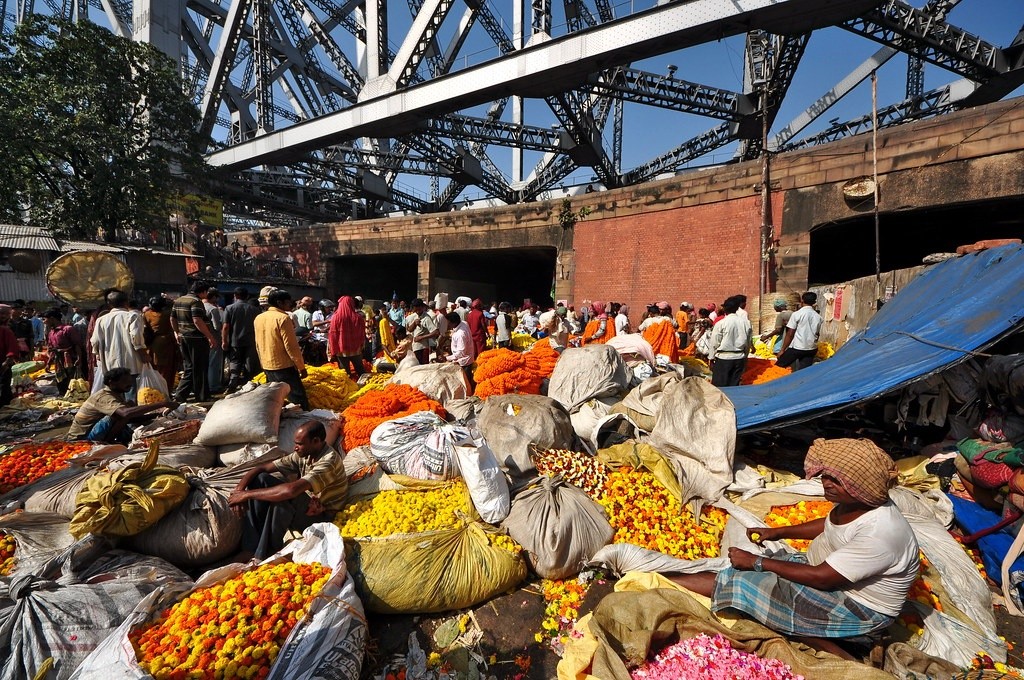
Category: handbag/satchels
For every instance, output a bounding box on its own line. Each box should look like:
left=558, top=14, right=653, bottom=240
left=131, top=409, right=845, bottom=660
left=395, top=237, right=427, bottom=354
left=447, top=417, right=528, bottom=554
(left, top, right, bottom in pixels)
left=136, top=362, right=172, bottom=415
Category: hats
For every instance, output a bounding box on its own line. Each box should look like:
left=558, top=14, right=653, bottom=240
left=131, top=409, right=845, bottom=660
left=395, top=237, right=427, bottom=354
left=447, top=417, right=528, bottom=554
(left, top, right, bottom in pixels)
left=658, top=302, right=669, bottom=309
left=681, top=302, right=689, bottom=307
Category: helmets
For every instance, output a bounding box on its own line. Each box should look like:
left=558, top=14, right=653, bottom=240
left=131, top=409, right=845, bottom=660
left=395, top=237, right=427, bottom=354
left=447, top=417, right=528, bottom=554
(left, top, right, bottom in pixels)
left=318, top=299, right=335, bottom=311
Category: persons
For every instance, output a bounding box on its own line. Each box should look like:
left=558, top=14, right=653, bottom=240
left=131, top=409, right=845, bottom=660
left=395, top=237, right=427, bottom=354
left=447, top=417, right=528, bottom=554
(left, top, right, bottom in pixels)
left=761, top=291, right=824, bottom=370
left=585, top=184, right=598, bottom=193
left=346, top=212, right=353, bottom=221
left=228, top=420, right=348, bottom=567
left=86, top=279, right=409, bottom=413
left=451, top=205, right=459, bottom=212
left=97, top=220, right=294, bottom=277
left=461, top=202, right=470, bottom=210
left=0, top=299, right=89, bottom=410
left=67, top=367, right=180, bottom=447
left=636, top=295, right=757, bottom=387
left=659, top=438, right=921, bottom=640
left=538, top=301, right=634, bottom=348
left=404, top=292, right=543, bottom=394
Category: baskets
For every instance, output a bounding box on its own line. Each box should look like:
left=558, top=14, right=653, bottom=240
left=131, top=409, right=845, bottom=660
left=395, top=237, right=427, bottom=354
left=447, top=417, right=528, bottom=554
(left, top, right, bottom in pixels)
left=152, top=419, right=200, bottom=447
